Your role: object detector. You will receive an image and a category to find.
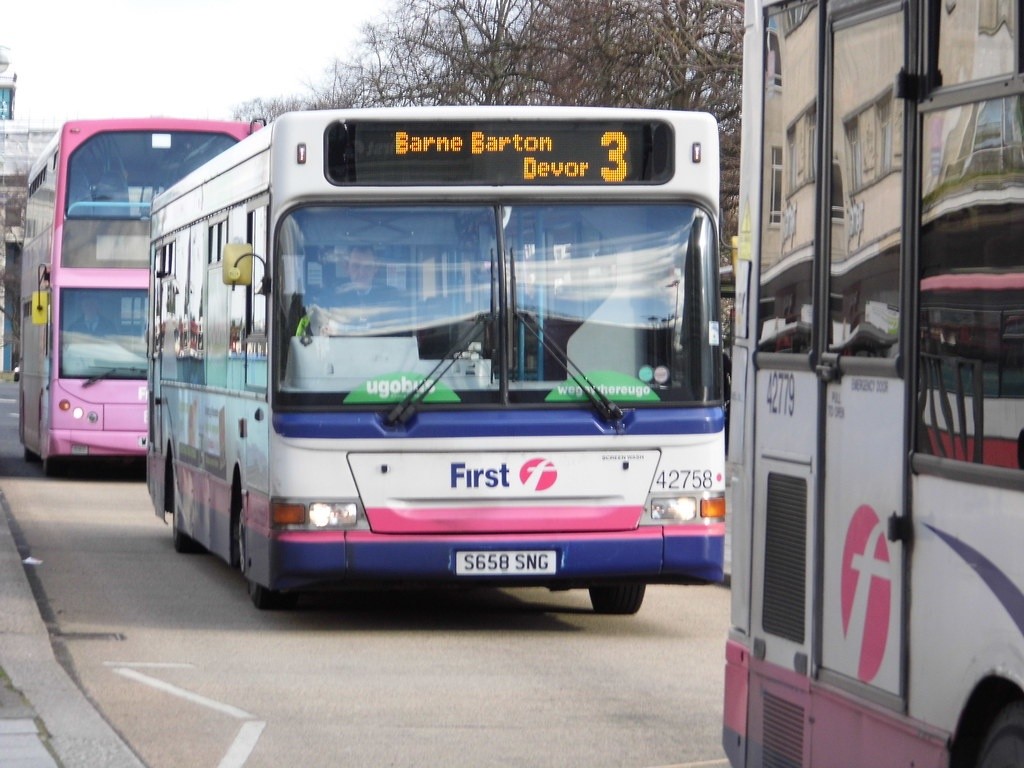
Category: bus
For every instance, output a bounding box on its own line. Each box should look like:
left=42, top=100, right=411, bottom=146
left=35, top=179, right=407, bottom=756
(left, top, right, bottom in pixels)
left=19, top=118, right=269, bottom=478
left=723, top=3, right=1024, bottom=767
left=146, top=105, right=732, bottom=617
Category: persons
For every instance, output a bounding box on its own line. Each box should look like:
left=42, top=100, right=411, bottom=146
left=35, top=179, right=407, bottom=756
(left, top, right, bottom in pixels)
left=65, top=294, right=117, bottom=335
left=317, top=243, right=411, bottom=309
